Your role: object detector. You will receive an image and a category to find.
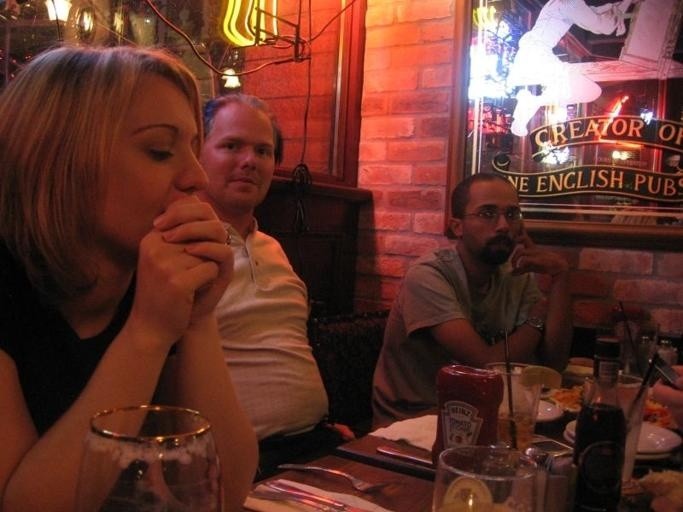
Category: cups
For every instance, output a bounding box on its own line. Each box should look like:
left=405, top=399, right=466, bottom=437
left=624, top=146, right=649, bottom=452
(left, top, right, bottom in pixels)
left=581, top=371, right=649, bottom=487
left=613, top=318, right=661, bottom=381
left=479, top=360, right=546, bottom=455
left=70, top=403, right=222, bottom=511
left=430, top=442, right=539, bottom=512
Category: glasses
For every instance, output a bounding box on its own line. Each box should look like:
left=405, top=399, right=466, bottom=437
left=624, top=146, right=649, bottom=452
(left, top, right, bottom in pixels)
left=464, top=210, right=523, bottom=223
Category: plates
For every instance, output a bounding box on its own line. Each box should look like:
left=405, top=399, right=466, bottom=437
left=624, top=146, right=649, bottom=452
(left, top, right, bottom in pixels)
left=562, top=410, right=680, bottom=463
left=535, top=394, right=566, bottom=422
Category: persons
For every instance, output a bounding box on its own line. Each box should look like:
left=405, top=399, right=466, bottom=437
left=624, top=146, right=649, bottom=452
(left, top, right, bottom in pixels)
left=366, top=173, right=572, bottom=433
left=200, top=94, right=355, bottom=483
left=506, top=0, right=625, bottom=138
left=0, top=42, right=260, bottom=512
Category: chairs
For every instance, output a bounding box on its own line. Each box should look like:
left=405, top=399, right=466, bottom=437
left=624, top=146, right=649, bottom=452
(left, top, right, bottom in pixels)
left=305, top=310, right=392, bottom=440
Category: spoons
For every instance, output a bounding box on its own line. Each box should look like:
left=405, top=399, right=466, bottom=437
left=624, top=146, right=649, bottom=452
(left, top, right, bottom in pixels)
left=275, top=456, right=393, bottom=493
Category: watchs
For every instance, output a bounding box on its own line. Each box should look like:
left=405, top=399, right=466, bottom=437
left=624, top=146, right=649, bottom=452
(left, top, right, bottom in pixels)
left=524, top=317, right=544, bottom=334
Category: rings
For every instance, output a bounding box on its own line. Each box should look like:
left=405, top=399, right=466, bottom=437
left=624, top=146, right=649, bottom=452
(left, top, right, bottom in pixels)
left=223, top=226, right=234, bottom=246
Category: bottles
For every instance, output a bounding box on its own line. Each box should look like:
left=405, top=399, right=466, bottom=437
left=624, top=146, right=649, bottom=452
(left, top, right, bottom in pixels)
left=430, top=366, right=503, bottom=468
left=574, top=336, right=628, bottom=506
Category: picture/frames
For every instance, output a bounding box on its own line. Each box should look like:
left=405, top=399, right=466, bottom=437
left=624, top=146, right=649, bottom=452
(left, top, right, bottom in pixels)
left=444, top=0, right=683, bottom=241
